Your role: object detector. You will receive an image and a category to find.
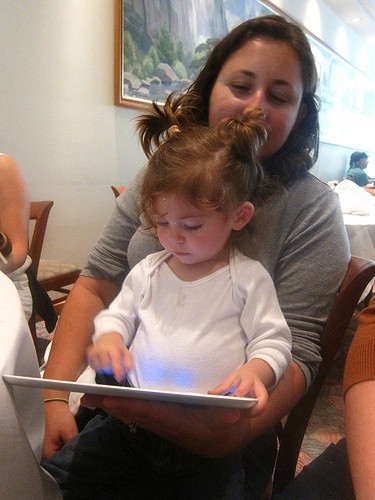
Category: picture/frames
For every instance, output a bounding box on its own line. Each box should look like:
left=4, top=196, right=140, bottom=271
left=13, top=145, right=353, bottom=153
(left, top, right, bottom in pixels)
left=113, top=0, right=288, bottom=118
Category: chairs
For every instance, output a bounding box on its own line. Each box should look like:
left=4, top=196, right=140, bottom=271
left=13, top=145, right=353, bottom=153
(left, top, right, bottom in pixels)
left=28, top=200, right=375, bottom=500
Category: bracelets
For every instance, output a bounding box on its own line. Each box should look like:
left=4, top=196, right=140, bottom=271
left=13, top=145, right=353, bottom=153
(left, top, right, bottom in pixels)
left=42, top=397, right=72, bottom=405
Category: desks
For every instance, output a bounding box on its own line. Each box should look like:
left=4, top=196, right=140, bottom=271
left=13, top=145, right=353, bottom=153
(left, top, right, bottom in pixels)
left=330, top=180, right=375, bottom=304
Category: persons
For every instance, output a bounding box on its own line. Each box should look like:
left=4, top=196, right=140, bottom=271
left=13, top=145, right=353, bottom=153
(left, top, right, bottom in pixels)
left=86, top=85, right=294, bottom=499
left=39, top=14, right=352, bottom=500
left=0, top=152, right=33, bottom=324
left=346, top=151, right=372, bottom=186
left=289, top=270, right=374, bottom=500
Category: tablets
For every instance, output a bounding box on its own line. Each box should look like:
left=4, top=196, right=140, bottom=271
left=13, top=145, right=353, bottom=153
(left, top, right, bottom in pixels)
left=2, top=373, right=258, bottom=411
